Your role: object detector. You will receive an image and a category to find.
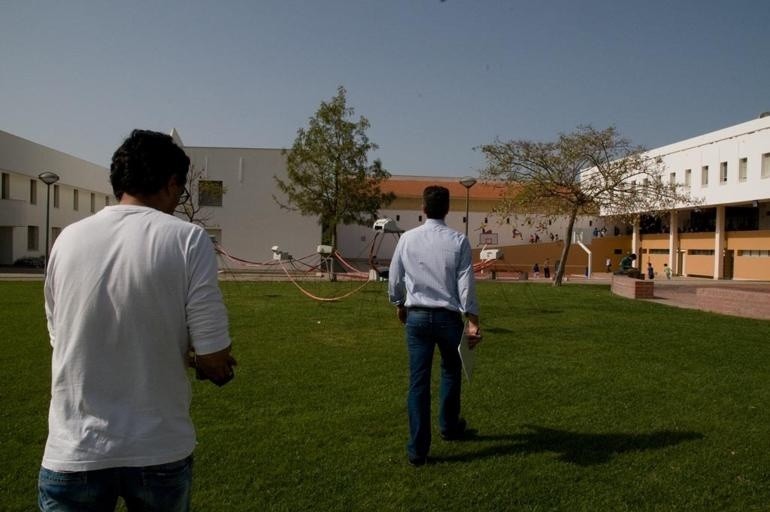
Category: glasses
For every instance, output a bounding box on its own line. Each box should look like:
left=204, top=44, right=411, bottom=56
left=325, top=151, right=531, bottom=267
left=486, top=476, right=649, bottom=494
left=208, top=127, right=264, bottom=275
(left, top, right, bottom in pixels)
left=176, top=185, right=190, bottom=204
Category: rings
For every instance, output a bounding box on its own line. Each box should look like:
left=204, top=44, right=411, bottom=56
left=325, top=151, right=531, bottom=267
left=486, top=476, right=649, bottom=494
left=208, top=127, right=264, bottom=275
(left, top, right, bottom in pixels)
left=229, top=371, right=233, bottom=376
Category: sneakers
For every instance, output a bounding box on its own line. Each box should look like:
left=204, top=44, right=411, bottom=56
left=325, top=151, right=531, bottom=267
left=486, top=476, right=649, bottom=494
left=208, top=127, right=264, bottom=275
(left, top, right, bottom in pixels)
left=408, top=454, right=426, bottom=466
left=440, top=418, right=466, bottom=441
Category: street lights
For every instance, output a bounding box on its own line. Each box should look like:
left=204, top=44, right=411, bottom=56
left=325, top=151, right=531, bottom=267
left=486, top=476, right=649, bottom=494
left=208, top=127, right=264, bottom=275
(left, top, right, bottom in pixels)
left=38, top=172, right=60, bottom=278
left=459, top=176, right=477, bottom=236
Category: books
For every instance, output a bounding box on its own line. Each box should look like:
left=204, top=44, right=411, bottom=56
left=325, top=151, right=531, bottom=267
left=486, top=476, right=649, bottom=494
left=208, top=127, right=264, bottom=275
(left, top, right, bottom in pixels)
left=457, top=319, right=477, bottom=386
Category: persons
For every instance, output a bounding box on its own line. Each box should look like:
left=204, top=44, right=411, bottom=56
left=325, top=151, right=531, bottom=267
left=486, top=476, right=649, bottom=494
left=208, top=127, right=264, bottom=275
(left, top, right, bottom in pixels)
left=534, top=232, right=540, bottom=243
left=584, top=266, right=588, bottom=279
left=647, top=262, right=654, bottom=279
left=555, top=234, right=559, bottom=241
left=529, top=234, right=535, bottom=243
left=600, top=226, right=608, bottom=237
left=592, top=227, right=600, bottom=237
left=624, top=221, right=718, bottom=235
left=663, top=264, right=673, bottom=280
left=36, top=127, right=240, bottom=511
left=386, top=183, right=485, bottom=467
left=532, top=263, right=540, bottom=278
left=606, top=256, right=612, bottom=273
left=550, top=232, right=554, bottom=241
left=618, top=253, right=637, bottom=271
left=543, top=257, right=550, bottom=278
